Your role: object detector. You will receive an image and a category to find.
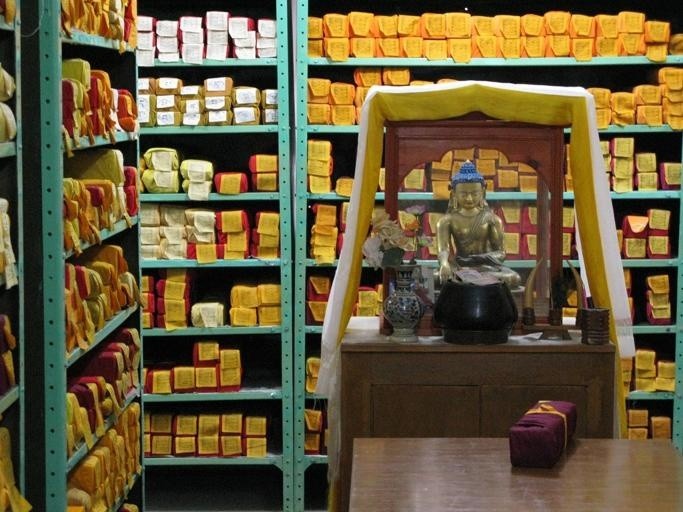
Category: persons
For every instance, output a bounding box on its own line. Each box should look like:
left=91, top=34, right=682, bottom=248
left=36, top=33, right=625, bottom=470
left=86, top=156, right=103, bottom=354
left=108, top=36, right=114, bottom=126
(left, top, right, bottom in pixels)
left=431, top=160, right=521, bottom=296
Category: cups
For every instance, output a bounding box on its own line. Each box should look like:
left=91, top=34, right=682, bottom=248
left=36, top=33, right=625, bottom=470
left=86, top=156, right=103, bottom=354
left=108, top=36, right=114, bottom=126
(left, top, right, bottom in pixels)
left=581, top=306, right=609, bottom=345
